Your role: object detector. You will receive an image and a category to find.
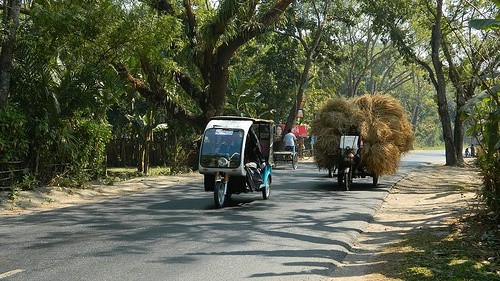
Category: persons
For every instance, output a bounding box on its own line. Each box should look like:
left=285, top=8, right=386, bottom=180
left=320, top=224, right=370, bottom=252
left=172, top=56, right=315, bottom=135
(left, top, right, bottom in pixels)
left=342, top=126, right=363, bottom=174
left=284, top=129, right=315, bottom=158
left=276, top=125, right=283, bottom=135
left=465, top=144, right=480, bottom=157
left=218, top=134, right=240, bottom=167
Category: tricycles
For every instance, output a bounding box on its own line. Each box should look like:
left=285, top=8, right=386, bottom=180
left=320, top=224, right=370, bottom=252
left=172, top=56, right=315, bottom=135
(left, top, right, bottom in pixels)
left=198, top=115, right=273, bottom=208
left=272, top=146, right=298, bottom=170
left=328, top=147, right=379, bottom=190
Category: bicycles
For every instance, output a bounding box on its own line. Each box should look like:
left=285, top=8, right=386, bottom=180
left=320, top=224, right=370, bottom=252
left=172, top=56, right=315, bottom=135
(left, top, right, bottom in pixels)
left=297, top=148, right=311, bottom=160
